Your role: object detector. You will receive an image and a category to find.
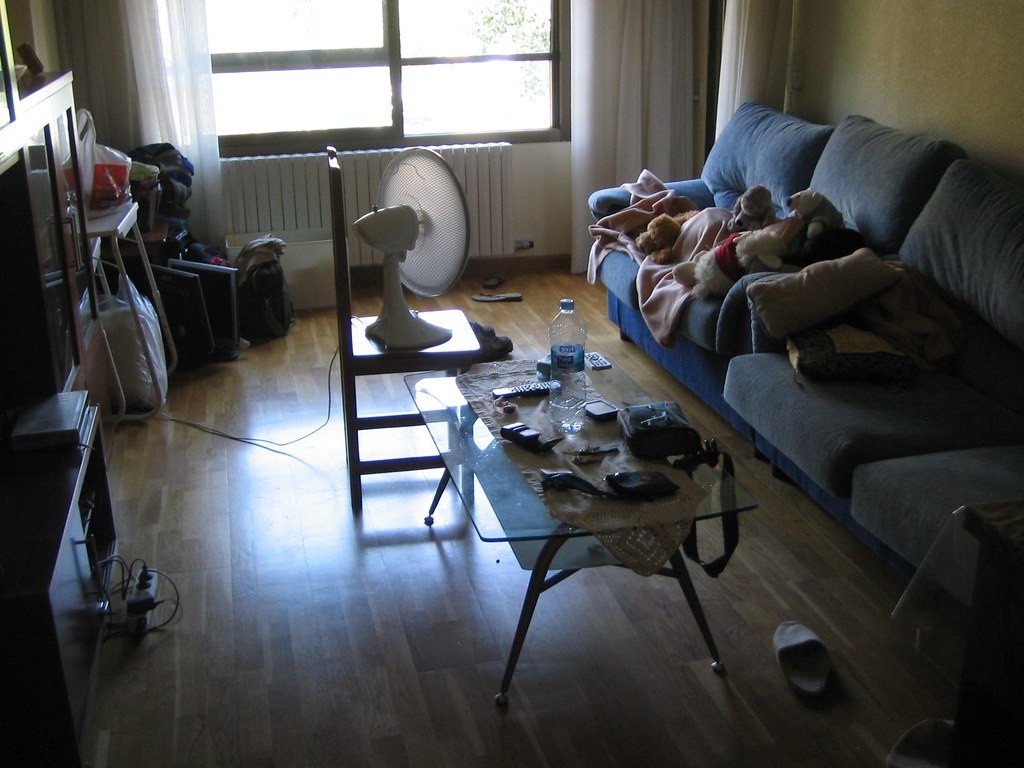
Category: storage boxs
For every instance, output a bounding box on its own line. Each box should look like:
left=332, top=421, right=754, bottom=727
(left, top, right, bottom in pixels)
left=227, top=229, right=335, bottom=313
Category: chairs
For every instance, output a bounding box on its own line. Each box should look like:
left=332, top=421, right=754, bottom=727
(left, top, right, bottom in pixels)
left=321, top=144, right=514, bottom=513
left=957, top=506, right=1024, bottom=768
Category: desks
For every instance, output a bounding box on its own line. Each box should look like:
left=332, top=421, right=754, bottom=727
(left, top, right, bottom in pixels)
left=86, top=202, right=172, bottom=423
left=102, top=223, right=172, bottom=261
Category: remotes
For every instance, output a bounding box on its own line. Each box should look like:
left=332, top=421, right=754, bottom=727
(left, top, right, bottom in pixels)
left=492, top=380, right=563, bottom=399
left=583, top=352, right=612, bottom=370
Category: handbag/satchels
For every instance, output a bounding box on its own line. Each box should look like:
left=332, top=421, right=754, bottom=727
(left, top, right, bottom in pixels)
left=234, top=260, right=292, bottom=344
left=618, top=402, right=701, bottom=458
left=54, top=105, right=132, bottom=219
left=71, top=271, right=169, bottom=416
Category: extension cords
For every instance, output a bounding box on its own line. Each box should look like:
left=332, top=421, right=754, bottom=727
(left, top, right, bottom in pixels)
left=127, top=568, right=158, bottom=614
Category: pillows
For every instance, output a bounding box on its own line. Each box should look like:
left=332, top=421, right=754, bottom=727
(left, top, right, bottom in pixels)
left=809, top=112, right=965, bottom=252
left=704, top=102, right=834, bottom=225
left=898, top=156, right=1024, bottom=341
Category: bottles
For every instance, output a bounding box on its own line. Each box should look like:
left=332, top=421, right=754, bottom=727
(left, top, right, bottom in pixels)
left=548, top=299, right=587, bottom=434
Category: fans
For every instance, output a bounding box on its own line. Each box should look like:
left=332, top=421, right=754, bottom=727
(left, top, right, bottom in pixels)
left=353, top=146, right=468, bottom=348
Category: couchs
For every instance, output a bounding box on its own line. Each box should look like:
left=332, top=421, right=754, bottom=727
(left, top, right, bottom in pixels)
left=588, top=100, right=1024, bottom=596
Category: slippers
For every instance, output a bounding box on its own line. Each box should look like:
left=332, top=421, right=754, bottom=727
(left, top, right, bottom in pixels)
left=483, top=272, right=505, bottom=288
left=472, top=291, right=522, bottom=302
left=887, top=719, right=956, bottom=768
left=772, top=620, right=831, bottom=695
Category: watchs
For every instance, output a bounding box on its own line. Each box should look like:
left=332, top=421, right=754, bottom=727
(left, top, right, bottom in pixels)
left=494, top=396, right=518, bottom=414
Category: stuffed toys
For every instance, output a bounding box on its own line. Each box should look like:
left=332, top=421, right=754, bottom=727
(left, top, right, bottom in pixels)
left=635, top=184, right=845, bottom=303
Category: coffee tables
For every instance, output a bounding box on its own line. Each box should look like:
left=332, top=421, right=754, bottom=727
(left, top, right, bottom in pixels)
left=402, top=362, right=757, bottom=707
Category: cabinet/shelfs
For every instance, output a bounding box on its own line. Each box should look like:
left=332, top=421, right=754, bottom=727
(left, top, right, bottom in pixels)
left=0, top=0, right=118, bottom=768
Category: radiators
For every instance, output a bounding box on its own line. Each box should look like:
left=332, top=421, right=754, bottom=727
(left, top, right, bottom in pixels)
left=219, top=143, right=515, bottom=263
